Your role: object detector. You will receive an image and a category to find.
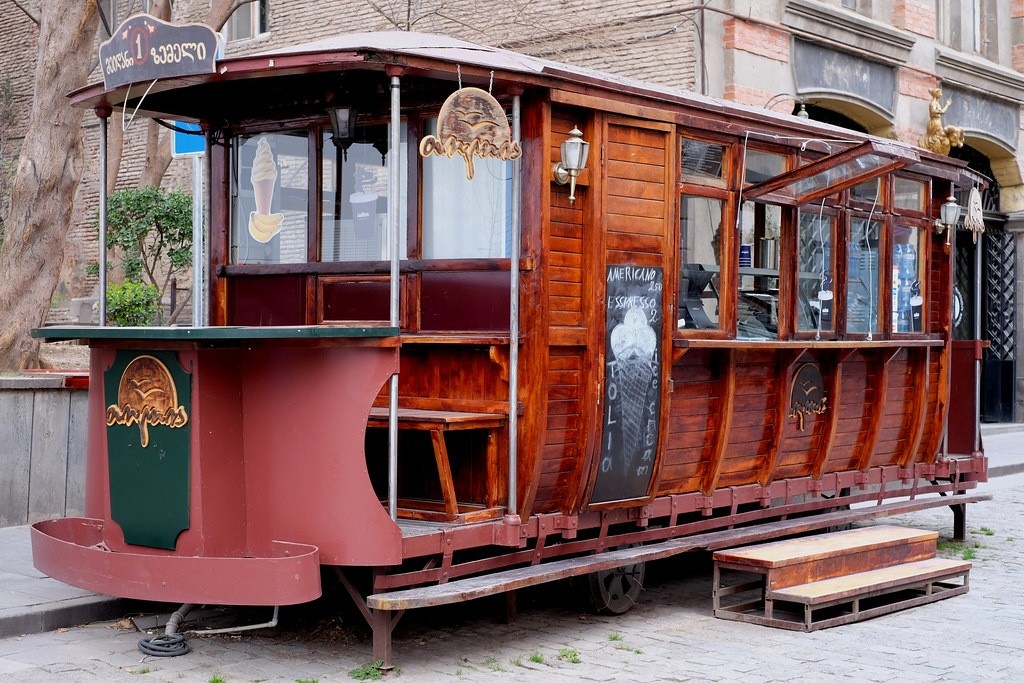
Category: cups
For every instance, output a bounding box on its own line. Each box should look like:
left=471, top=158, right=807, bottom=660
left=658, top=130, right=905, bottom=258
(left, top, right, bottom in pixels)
left=349, top=192, right=378, bottom=239
left=739, top=244, right=751, bottom=267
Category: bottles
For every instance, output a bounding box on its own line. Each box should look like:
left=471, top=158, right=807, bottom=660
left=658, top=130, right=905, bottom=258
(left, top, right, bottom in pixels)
left=824, top=241, right=861, bottom=275
left=894, top=244, right=916, bottom=332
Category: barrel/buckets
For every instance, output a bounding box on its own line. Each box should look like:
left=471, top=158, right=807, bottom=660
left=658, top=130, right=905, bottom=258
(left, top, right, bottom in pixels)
left=760, top=240, right=778, bottom=269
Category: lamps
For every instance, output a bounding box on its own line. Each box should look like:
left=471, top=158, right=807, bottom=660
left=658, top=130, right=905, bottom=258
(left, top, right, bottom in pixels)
left=935, top=194, right=961, bottom=247
left=553, top=124, right=589, bottom=204
left=324, top=84, right=359, bottom=162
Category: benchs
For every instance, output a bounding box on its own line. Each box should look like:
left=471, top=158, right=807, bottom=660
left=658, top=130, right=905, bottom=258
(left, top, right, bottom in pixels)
left=365, top=397, right=505, bottom=525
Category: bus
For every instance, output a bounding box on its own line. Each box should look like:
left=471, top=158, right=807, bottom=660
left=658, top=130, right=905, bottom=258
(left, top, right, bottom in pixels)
left=24, top=11, right=994, bottom=674
